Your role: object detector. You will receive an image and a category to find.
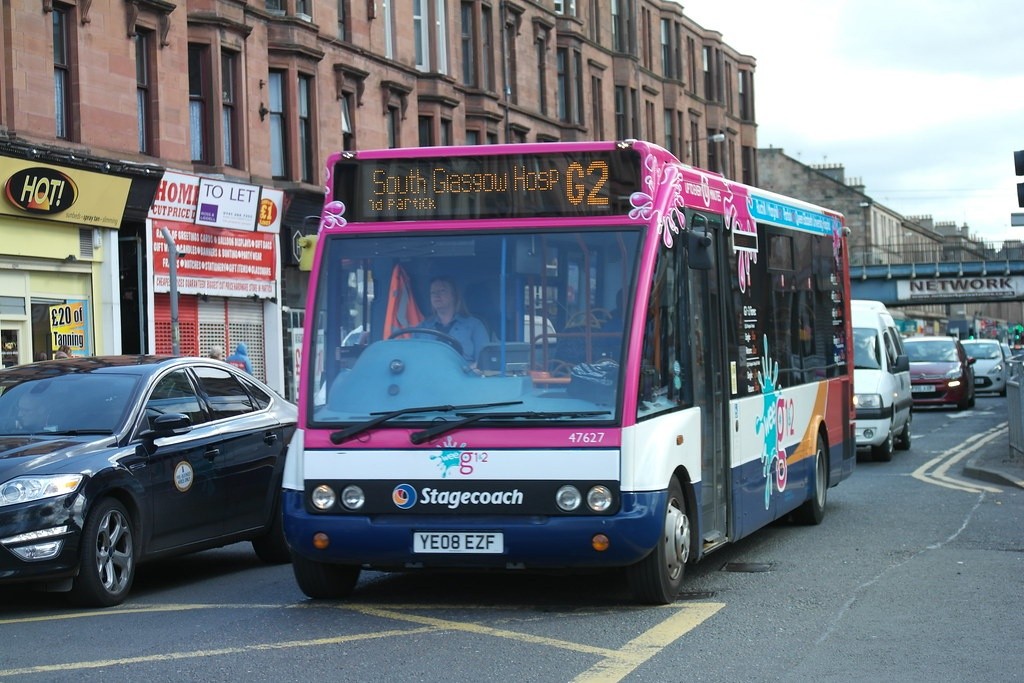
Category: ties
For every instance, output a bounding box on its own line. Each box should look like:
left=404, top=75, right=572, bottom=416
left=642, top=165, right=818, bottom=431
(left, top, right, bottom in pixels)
left=434, top=320, right=457, bottom=344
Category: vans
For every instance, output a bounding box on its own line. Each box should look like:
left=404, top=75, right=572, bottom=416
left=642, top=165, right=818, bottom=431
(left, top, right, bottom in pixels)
left=851, top=300, right=914, bottom=463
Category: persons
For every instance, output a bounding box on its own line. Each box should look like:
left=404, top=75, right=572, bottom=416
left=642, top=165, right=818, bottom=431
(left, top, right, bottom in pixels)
left=602, top=286, right=624, bottom=363
left=226, top=343, right=252, bottom=375
left=209, top=346, right=223, bottom=361
left=38, top=345, right=72, bottom=361
left=410, top=275, right=491, bottom=375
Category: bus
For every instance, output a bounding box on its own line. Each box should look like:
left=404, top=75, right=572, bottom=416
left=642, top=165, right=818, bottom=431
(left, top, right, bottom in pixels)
left=281, top=139, right=860, bottom=606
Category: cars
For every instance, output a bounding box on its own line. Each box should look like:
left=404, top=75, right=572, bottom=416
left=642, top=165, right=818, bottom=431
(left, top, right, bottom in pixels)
left=1001, top=342, right=1012, bottom=363
left=956, top=339, right=1008, bottom=398
left=0, top=351, right=298, bottom=610
left=900, top=335, right=977, bottom=412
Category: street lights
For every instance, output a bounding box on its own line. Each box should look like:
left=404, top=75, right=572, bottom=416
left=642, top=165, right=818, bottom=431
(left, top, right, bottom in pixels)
left=692, top=133, right=726, bottom=165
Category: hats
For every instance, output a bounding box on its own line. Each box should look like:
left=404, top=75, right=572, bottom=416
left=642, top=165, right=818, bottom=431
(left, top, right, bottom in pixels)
left=210, top=346, right=222, bottom=357
left=38, top=352, right=47, bottom=360
left=55, top=352, right=67, bottom=358
